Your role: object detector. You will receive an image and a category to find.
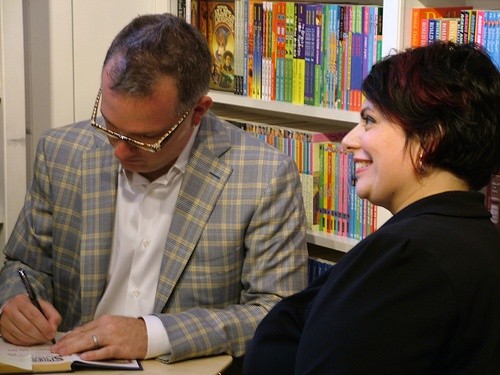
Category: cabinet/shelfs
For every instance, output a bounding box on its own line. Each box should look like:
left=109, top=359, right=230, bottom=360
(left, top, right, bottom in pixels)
left=156, top=0, right=500, bottom=286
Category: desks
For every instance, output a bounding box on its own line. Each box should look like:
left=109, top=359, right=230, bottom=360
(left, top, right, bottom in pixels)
left=0, top=352, right=233, bottom=375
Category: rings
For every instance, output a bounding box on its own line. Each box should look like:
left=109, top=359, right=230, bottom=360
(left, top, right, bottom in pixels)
left=92, top=335, right=98, bottom=347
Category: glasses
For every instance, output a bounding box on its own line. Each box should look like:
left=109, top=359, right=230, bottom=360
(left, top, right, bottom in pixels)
left=90, top=87, right=196, bottom=153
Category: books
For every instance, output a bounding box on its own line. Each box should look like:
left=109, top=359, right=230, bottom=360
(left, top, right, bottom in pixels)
left=483, top=168, right=500, bottom=226
left=0, top=331, right=143, bottom=375
left=220, top=115, right=377, bottom=241
left=306, top=251, right=340, bottom=285
left=185, top=0, right=500, bottom=114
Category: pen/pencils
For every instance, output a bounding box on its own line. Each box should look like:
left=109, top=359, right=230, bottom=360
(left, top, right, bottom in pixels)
left=17, top=267, right=57, bottom=345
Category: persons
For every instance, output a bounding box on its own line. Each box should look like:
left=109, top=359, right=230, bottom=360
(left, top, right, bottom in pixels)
left=216, top=38, right=500, bottom=375
left=0, top=13, right=309, bottom=362
